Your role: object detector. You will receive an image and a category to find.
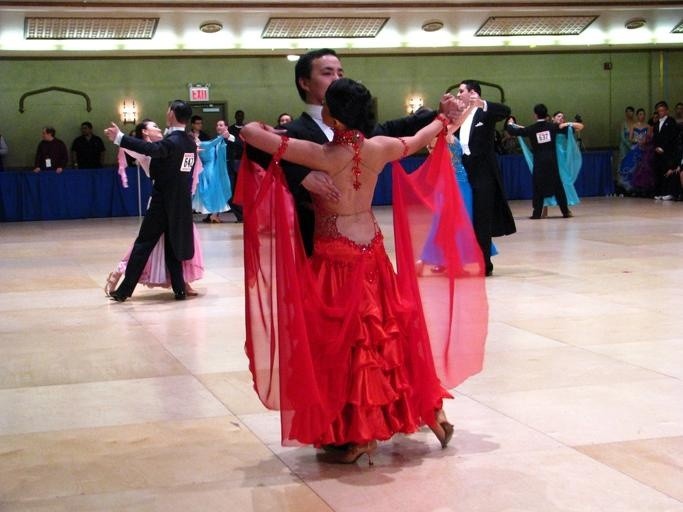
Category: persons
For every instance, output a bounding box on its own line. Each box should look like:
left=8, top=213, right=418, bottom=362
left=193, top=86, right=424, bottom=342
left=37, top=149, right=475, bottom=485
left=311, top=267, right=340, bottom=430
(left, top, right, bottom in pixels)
left=420, top=79, right=585, bottom=278
left=0, top=134, right=9, bottom=155
left=103, top=119, right=203, bottom=298
left=615, top=101, right=683, bottom=201
left=102, top=98, right=197, bottom=302
left=187, top=108, right=245, bottom=223
left=230, top=78, right=490, bottom=468
left=124, top=130, right=138, bottom=166
left=279, top=112, right=292, bottom=126
left=247, top=46, right=474, bottom=456
left=69, top=121, right=106, bottom=169
left=32, top=127, right=68, bottom=174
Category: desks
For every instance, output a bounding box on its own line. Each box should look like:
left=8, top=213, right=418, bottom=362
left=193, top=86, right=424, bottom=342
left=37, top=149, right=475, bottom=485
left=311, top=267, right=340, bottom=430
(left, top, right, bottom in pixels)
left=0, top=148, right=615, bottom=223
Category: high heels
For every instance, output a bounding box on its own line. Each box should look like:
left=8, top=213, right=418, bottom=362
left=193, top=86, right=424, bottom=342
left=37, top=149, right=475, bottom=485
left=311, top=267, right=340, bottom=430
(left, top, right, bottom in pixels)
left=316, top=437, right=377, bottom=467
left=415, top=260, right=425, bottom=277
left=428, top=410, right=455, bottom=449
left=210, top=214, right=225, bottom=223
left=185, top=285, right=198, bottom=296
left=104, top=271, right=118, bottom=296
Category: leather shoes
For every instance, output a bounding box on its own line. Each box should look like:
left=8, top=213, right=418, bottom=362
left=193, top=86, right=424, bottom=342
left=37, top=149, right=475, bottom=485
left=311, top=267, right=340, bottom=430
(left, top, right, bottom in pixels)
left=432, top=265, right=444, bottom=274
left=175, top=291, right=185, bottom=300
left=563, top=213, right=569, bottom=218
left=479, top=270, right=493, bottom=276
left=109, top=290, right=126, bottom=302
left=528, top=215, right=540, bottom=218
